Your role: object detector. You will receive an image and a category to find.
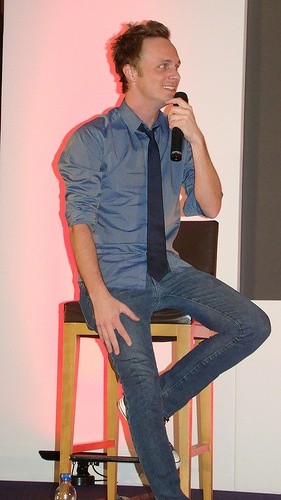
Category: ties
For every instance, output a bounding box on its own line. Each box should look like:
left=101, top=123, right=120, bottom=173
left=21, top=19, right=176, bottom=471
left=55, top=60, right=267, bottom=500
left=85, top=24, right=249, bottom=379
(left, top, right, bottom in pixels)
left=137, top=124, right=171, bottom=283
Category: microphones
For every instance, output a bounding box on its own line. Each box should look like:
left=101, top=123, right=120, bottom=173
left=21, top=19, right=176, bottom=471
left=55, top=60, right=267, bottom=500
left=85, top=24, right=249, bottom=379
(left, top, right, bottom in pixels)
left=170, top=91, right=188, bottom=162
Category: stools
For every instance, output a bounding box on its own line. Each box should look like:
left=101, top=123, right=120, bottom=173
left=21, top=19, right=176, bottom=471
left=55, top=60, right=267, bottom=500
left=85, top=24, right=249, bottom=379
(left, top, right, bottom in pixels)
left=59, top=220, right=219, bottom=500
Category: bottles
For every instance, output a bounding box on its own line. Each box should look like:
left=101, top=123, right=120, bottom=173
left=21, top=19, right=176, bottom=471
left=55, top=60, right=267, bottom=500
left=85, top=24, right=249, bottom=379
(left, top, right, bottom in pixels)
left=54, top=473, right=77, bottom=500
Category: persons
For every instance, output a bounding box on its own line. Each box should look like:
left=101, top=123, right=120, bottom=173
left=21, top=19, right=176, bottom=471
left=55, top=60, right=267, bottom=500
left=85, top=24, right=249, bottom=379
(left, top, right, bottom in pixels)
left=54, top=20, right=272, bottom=500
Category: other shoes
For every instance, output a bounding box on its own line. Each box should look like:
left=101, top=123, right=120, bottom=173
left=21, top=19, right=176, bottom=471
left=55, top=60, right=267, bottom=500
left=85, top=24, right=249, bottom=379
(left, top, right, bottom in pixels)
left=116, top=397, right=181, bottom=468
